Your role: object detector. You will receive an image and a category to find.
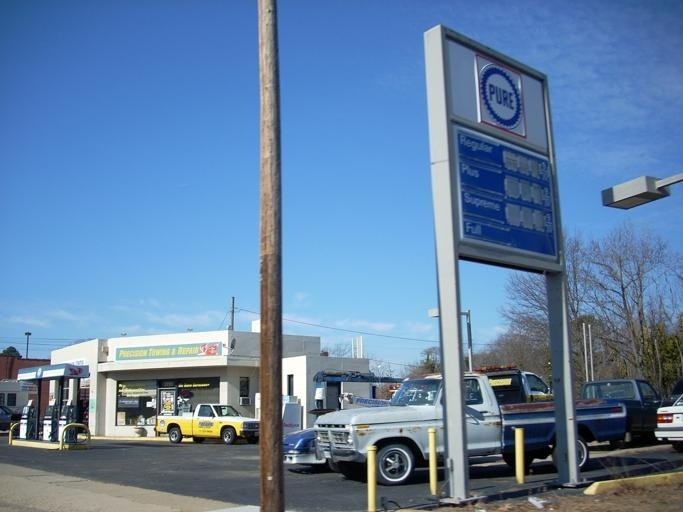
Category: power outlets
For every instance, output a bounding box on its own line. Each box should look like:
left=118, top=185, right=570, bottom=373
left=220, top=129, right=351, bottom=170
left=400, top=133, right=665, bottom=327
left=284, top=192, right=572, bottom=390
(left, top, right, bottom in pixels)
left=154, top=403, right=261, bottom=445
left=312, top=371, right=627, bottom=487
left=473, top=363, right=677, bottom=452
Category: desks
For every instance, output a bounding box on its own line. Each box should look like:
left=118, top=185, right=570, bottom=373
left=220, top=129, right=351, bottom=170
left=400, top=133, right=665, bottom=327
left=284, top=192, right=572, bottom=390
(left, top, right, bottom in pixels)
left=427, top=307, right=475, bottom=373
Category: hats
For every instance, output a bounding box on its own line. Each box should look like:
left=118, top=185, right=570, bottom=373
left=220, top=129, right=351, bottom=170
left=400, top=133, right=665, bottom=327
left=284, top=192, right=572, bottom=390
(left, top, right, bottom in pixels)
left=654, top=393, right=683, bottom=454
